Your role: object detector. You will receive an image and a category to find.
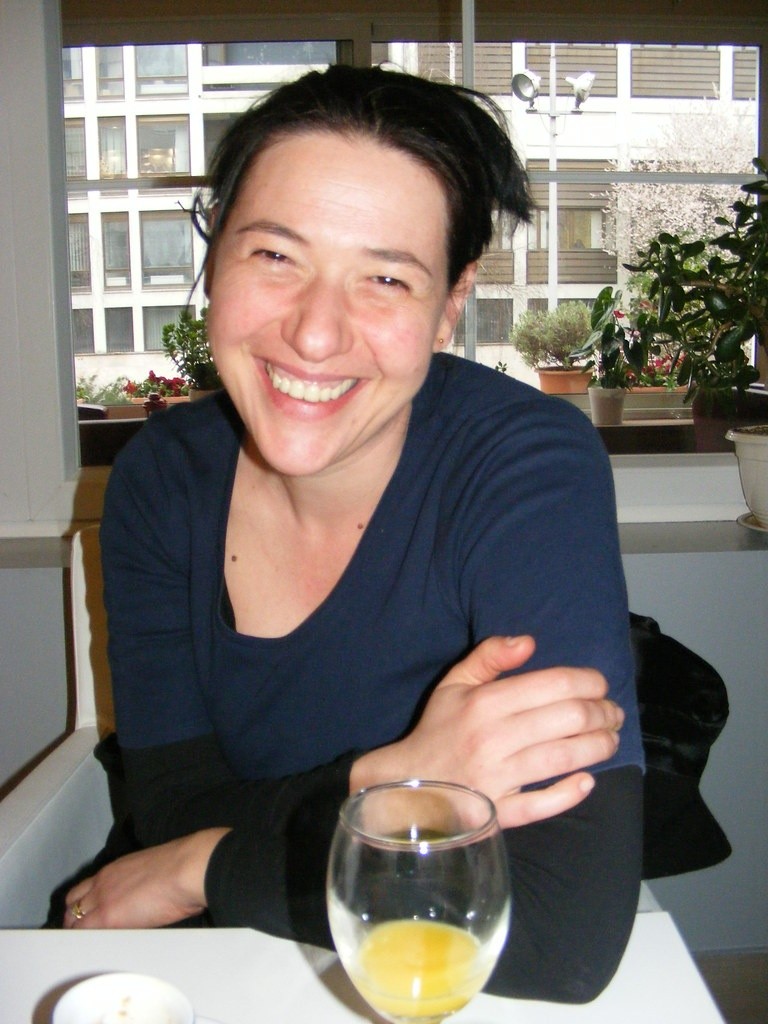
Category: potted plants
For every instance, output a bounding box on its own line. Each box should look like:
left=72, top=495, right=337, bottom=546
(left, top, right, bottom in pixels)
left=509, top=156, right=768, bottom=532
left=161, top=303, right=226, bottom=402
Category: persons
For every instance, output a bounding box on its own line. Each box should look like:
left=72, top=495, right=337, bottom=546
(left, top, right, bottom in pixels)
left=61, top=65, right=642, bottom=1006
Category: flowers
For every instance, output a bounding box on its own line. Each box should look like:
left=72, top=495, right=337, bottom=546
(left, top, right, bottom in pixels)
left=122, top=370, right=186, bottom=398
left=639, top=354, right=685, bottom=389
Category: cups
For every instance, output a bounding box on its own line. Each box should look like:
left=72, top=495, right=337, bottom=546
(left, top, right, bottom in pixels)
left=327, top=781, right=512, bottom=1024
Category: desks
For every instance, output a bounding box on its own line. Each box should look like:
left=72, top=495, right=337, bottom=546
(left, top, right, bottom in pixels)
left=0, top=910, right=728, bottom=1024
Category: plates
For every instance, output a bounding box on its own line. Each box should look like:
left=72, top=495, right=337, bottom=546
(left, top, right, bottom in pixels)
left=51, top=973, right=194, bottom=1024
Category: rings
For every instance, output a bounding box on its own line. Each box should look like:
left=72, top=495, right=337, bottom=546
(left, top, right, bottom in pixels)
left=71, top=900, right=85, bottom=919
